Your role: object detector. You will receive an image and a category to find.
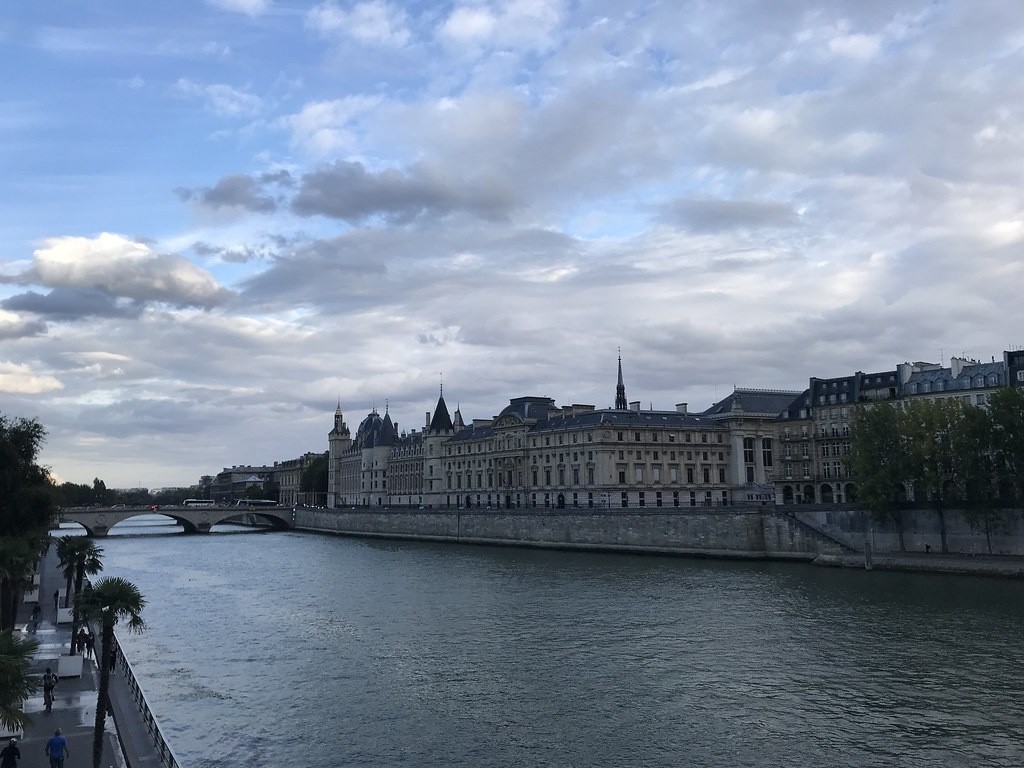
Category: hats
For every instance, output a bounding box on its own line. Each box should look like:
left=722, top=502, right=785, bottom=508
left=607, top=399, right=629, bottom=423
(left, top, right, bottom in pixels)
left=56, top=728, right=62, bottom=734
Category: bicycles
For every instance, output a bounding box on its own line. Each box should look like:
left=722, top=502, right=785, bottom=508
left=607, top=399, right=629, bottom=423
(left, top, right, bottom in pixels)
left=40, top=681, right=57, bottom=713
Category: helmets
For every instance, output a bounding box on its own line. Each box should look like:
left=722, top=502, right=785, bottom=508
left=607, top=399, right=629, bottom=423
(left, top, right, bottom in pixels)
left=46, top=667, right=52, bottom=673
left=10, top=738, right=17, bottom=746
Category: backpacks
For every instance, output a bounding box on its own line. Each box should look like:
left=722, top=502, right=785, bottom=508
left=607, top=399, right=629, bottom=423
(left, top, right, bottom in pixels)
left=44, top=673, right=56, bottom=687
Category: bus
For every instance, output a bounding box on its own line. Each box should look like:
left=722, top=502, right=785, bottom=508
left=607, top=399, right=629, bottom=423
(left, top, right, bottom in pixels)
left=237, top=499, right=277, bottom=506
left=183, top=499, right=215, bottom=506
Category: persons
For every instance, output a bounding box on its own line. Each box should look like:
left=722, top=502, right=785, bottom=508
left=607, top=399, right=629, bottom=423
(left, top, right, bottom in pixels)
left=109, top=643, right=118, bottom=674
left=0, top=738, right=21, bottom=768
left=77, top=629, right=87, bottom=662
left=53, top=590, right=58, bottom=609
left=86, top=632, right=95, bottom=659
left=32, top=603, right=40, bottom=620
left=41, top=667, right=59, bottom=705
left=45, top=728, right=70, bottom=768
